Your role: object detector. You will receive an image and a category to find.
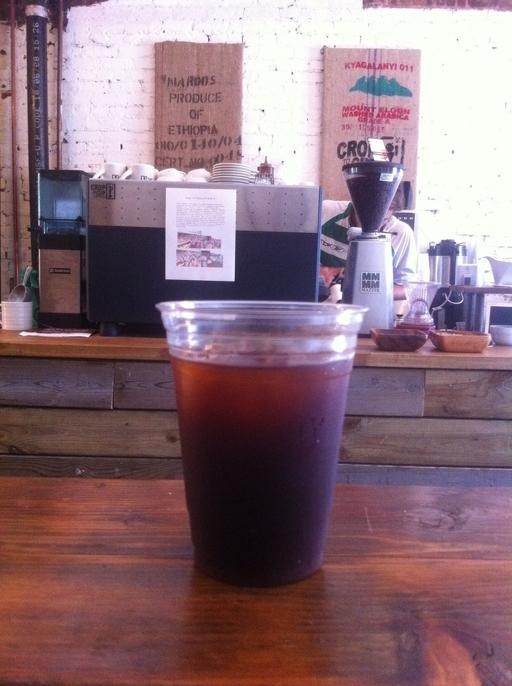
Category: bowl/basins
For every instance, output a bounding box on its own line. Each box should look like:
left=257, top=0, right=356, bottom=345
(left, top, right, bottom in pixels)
left=490, top=325, right=512, bottom=345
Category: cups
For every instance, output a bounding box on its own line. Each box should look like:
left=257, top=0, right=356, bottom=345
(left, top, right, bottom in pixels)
left=0, top=302, right=34, bottom=331
left=154, top=301, right=368, bottom=586
left=254, top=156, right=275, bottom=185
left=92, top=161, right=210, bottom=182
left=403, top=275, right=426, bottom=318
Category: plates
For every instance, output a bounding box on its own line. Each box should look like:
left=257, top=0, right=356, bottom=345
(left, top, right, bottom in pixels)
left=208, top=163, right=256, bottom=184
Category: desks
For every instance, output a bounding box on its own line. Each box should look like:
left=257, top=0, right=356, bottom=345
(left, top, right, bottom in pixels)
left=1, top=471, right=511, bottom=686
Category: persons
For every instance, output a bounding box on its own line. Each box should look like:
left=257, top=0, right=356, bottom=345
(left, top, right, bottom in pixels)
left=318, top=189, right=416, bottom=313
